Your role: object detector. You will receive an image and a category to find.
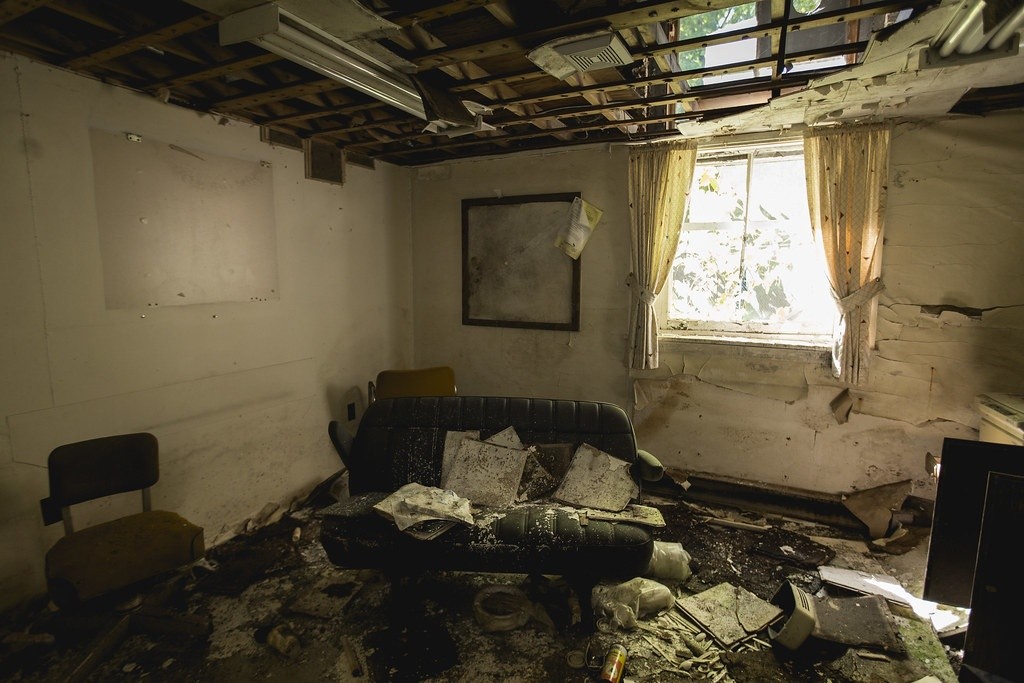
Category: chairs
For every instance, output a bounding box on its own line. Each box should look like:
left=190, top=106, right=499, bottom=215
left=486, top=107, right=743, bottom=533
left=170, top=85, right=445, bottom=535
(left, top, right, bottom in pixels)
left=367, top=366, right=458, bottom=405
left=47, top=433, right=212, bottom=683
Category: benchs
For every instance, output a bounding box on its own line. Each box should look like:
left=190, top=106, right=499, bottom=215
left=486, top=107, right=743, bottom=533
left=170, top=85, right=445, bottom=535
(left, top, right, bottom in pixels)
left=322, top=395, right=654, bottom=636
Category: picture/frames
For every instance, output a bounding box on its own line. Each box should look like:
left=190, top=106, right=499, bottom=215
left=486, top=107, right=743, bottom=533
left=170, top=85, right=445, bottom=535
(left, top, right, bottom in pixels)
left=460, top=191, right=582, bottom=332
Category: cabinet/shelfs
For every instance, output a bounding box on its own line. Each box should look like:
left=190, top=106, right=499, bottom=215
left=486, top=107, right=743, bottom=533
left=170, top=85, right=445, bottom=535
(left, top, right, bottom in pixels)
left=923, top=393, right=1024, bottom=683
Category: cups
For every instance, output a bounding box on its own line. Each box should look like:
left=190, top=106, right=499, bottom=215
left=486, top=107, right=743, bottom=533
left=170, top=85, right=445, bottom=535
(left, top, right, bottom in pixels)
left=268, top=624, right=299, bottom=655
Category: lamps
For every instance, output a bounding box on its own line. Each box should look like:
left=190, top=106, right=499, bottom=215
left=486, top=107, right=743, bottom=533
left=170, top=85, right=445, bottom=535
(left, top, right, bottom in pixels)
left=219, top=2, right=493, bottom=139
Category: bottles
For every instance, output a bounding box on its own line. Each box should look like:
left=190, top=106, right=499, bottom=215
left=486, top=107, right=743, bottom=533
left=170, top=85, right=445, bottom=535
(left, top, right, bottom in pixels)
left=292, top=527, right=300, bottom=541
left=599, top=641, right=628, bottom=683
